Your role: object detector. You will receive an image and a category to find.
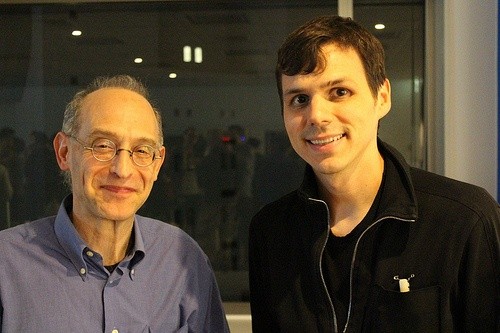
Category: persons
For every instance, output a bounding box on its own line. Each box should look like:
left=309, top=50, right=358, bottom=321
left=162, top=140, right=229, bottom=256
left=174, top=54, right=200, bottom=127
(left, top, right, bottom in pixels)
left=246, top=13, right=500, bottom=333
left=0, top=70, right=231, bottom=333
left=0, top=111, right=302, bottom=273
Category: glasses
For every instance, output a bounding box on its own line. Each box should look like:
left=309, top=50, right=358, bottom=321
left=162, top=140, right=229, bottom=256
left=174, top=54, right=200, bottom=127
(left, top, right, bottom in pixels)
left=62, top=133, right=162, bottom=166
